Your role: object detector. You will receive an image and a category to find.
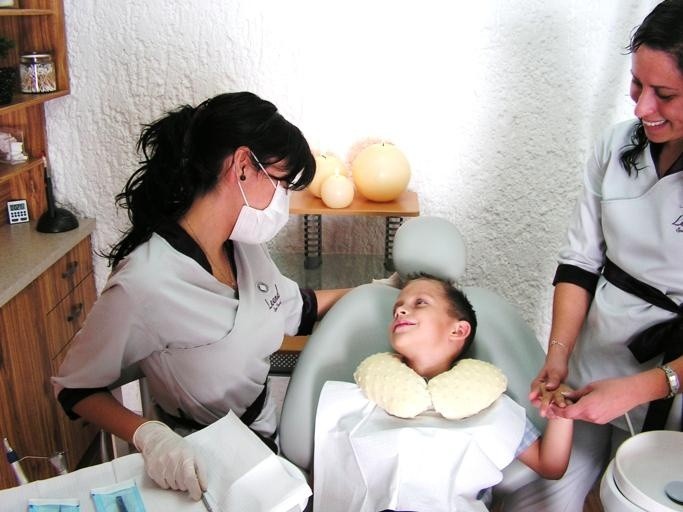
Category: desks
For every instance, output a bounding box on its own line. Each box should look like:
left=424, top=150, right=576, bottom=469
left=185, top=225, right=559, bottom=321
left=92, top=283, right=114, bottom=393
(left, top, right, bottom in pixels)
left=0, top=408, right=313, bottom=512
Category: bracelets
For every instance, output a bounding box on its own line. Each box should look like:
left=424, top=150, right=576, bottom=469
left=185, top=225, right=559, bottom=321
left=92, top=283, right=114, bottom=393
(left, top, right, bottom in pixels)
left=547, top=341, right=571, bottom=360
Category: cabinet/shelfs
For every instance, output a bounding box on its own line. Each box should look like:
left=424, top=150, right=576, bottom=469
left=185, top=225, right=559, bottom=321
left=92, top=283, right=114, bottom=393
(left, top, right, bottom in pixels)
left=0, top=0, right=100, bottom=493
left=267, top=189, right=420, bottom=377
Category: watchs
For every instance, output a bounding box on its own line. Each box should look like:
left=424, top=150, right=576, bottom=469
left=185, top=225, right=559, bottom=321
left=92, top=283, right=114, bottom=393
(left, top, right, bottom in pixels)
left=657, top=365, right=680, bottom=400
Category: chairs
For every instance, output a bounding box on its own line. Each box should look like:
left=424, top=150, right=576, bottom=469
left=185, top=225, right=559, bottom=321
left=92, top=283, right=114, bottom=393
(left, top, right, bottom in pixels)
left=278, top=215, right=548, bottom=496
left=97, top=359, right=157, bottom=464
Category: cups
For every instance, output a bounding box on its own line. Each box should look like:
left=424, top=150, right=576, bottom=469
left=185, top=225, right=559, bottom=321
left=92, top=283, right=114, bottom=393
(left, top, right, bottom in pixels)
left=0, top=125, right=26, bottom=165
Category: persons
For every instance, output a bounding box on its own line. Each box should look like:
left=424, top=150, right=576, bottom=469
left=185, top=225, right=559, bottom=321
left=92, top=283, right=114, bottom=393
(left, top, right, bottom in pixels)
left=311, top=268, right=579, bottom=512
left=53, top=90, right=406, bottom=502
left=498, top=0, right=682, bottom=512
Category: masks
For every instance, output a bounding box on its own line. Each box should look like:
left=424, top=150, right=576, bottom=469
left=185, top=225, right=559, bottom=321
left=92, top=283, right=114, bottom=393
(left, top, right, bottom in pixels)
left=225, top=180, right=292, bottom=246
